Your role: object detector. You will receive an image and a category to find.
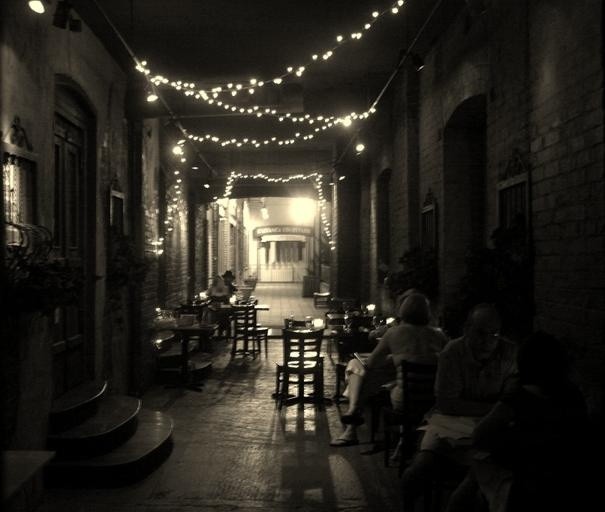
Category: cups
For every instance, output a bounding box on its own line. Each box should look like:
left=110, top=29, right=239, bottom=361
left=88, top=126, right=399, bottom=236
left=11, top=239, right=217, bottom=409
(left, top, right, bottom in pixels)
left=304, top=316, right=315, bottom=329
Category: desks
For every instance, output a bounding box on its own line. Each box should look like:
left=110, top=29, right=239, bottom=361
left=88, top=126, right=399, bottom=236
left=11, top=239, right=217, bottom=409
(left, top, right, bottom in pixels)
left=171, top=322, right=220, bottom=389
left=327, top=312, right=374, bottom=325
left=425, top=411, right=487, bottom=459
left=421, top=411, right=479, bottom=512
left=2, top=450, right=59, bottom=511
left=182, top=302, right=269, bottom=337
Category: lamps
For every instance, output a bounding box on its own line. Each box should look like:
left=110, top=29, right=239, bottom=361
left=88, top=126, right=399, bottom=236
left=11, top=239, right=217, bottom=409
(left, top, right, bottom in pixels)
left=407, top=54, right=427, bottom=73
left=174, top=127, right=186, bottom=144
left=144, top=85, right=158, bottom=102
left=354, top=130, right=364, bottom=154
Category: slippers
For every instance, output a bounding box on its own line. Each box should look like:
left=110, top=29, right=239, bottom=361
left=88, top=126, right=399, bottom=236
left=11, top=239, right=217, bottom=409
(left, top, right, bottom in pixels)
left=341, top=415, right=365, bottom=426
left=329, top=439, right=359, bottom=447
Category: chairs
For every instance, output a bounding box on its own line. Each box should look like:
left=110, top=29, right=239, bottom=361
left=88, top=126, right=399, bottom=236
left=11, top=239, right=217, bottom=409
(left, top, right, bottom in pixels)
left=284, top=319, right=317, bottom=328
left=335, top=341, right=362, bottom=401
left=272, top=329, right=332, bottom=409
left=231, top=305, right=268, bottom=359
left=234, top=275, right=257, bottom=300
left=382, top=361, right=437, bottom=479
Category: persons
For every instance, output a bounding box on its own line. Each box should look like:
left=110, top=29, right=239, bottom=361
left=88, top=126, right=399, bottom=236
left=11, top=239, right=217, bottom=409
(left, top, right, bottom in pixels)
left=364, top=292, right=452, bottom=462
left=446, top=326, right=592, bottom=511
left=206, top=270, right=238, bottom=344
left=200, top=274, right=229, bottom=327
left=328, top=285, right=420, bottom=448
left=398, top=301, right=528, bottom=511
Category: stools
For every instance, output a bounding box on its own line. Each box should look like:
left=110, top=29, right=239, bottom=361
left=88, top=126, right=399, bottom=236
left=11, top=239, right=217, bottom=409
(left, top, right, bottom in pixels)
left=313, top=292, right=330, bottom=306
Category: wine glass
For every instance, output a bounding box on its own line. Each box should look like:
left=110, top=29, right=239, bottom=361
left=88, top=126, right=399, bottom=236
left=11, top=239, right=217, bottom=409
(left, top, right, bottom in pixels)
left=344, top=314, right=353, bottom=332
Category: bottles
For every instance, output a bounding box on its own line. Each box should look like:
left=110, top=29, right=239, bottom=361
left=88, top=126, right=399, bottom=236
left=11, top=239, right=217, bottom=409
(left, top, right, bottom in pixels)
left=288, top=314, right=295, bottom=330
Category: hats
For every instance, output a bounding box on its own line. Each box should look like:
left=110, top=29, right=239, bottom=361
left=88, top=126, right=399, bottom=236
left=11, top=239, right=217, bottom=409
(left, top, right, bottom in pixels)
left=221, top=270, right=235, bottom=280
